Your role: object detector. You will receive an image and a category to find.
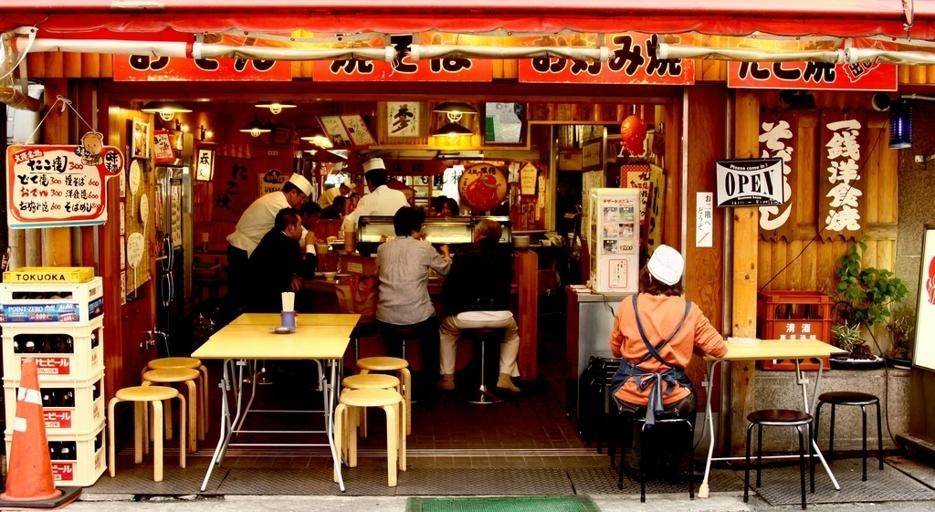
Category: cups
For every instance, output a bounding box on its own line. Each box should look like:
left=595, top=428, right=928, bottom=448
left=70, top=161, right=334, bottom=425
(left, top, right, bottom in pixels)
left=281, top=312, right=296, bottom=330
left=318, top=254, right=328, bottom=272
left=344, top=232, right=356, bottom=252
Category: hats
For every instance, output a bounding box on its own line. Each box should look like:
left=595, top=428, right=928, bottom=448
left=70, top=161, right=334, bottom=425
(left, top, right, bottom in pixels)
left=363, top=158, right=386, bottom=173
left=289, top=172, right=313, bottom=197
left=344, top=177, right=356, bottom=189
left=647, top=244, right=685, bottom=285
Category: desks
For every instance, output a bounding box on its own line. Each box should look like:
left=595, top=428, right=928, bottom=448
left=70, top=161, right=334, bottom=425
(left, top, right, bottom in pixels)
left=190, top=314, right=362, bottom=492
left=697, top=339, right=848, bottom=499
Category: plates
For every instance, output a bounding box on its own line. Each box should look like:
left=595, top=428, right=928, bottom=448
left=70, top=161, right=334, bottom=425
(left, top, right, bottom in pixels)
left=271, top=327, right=294, bottom=334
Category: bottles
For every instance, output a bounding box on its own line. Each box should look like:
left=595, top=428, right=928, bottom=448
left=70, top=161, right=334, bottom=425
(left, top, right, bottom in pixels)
left=10, top=292, right=99, bottom=460
left=775, top=304, right=814, bottom=319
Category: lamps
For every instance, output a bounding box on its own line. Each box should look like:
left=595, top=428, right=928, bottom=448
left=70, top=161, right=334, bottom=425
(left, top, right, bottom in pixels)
left=433, top=102, right=477, bottom=124
left=239, top=115, right=271, bottom=137
left=140, top=103, right=193, bottom=122
left=255, top=102, right=296, bottom=115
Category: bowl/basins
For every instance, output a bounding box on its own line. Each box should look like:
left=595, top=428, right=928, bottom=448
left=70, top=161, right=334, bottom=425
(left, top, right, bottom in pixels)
left=323, top=272, right=336, bottom=280
left=336, top=274, right=352, bottom=284
left=329, top=242, right=343, bottom=250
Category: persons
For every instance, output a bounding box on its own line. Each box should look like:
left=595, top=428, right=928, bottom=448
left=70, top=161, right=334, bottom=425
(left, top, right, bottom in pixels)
left=316, top=177, right=356, bottom=210
left=318, top=196, right=345, bottom=220
left=295, top=201, right=322, bottom=233
left=224, top=172, right=318, bottom=278
left=342, top=157, right=412, bottom=242
left=434, top=219, right=523, bottom=394
left=608, top=243, right=727, bottom=476
left=235, top=207, right=320, bottom=317
left=371, top=206, right=447, bottom=384
left=441, top=198, right=458, bottom=218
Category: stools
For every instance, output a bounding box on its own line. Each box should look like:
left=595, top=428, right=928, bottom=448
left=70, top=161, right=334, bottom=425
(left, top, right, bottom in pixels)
left=390, top=328, right=420, bottom=404
left=142, top=369, right=204, bottom=453
left=815, top=392, right=883, bottom=482
left=143, top=357, right=208, bottom=440
left=465, top=329, right=505, bottom=405
left=333, top=389, right=406, bottom=486
left=618, top=415, right=694, bottom=502
left=357, top=357, right=414, bottom=434
left=343, top=375, right=400, bottom=467
left=578, top=354, right=624, bottom=454
left=744, top=410, right=819, bottom=510
left=108, top=387, right=187, bottom=482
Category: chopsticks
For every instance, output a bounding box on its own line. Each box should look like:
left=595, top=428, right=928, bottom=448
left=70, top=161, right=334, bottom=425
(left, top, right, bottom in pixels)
left=281, top=292, right=295, bottom=312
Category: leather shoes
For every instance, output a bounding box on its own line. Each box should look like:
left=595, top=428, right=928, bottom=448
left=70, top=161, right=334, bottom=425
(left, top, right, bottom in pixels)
left=439, top=381, right=454, bottom=390
left=497, top=378, right=520, bottom=392
